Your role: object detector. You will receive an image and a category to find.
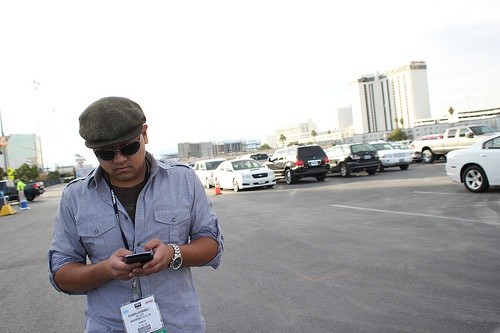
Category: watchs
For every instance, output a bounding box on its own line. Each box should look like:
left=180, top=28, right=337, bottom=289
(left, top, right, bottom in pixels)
left=164, top=242, right=183, bottom=273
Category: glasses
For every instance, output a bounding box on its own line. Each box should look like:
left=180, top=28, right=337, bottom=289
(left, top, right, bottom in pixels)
left=93, top=134, right=142, bottom=161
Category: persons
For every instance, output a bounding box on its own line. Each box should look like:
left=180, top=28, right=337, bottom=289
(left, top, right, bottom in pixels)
left=15, top=179, right=27, bottom=201
left=48, top=95, right=225, bottom=333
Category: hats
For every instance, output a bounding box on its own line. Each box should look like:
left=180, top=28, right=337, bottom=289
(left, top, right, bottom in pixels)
left=78, top=96, right=146, bottom=148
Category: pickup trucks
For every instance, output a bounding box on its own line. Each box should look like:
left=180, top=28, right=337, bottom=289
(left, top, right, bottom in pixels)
left=0, top=179, right=45, bottom=201
left=411, top=125, right=496, bottom=163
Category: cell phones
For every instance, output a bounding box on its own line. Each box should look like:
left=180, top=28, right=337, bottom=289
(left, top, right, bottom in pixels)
left=125, top=252, right=153, bottom=265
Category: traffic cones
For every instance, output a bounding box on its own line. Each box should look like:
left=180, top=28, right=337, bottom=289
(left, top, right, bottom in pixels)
left=214, top=177, right=222, bottom=195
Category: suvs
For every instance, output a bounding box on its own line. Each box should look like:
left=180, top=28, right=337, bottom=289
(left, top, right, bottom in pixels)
left=324, top=143, right=380, bottom=177
left=264, top=144, right=330, bottom=184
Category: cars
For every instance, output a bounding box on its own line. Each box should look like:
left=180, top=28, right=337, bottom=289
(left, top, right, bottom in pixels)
left=445, top=133, right=500, bottom=192
left=192, top=151, right=276, bottom=192
left=368, top=141, right=414, bottom=172
